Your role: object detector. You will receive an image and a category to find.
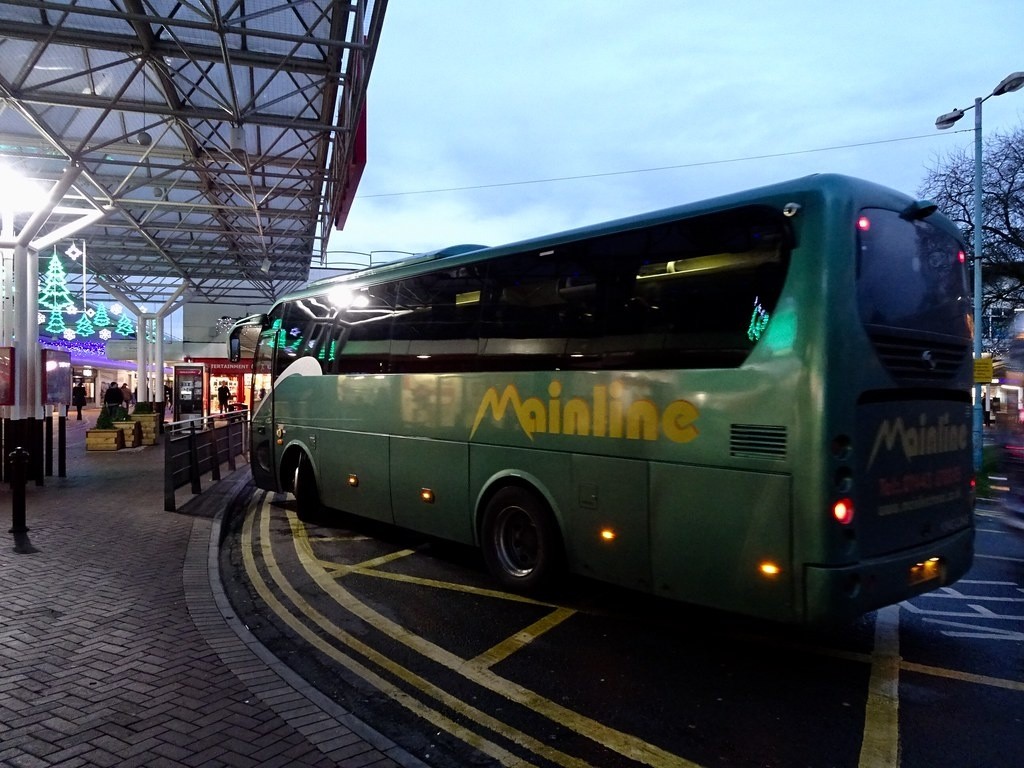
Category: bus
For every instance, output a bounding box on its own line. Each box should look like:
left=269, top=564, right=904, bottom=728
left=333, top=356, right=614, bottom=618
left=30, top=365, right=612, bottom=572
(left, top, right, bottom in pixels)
left=226, top=174, right=976, bottom=628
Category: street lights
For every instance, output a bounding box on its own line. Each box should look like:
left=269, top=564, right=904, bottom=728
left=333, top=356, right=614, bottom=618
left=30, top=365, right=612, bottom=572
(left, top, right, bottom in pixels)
left=933, top=73, right=1024, bottom=498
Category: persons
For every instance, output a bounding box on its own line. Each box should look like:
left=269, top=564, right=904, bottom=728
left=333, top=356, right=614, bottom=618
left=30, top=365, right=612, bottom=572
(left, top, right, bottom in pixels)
left=168, top=387, right=174, bottom=410
left=120, top=383, right=131, bottom=415
left=218, top=381, right=231, bottom=414
left=104, top=382, right=124, bottom=418
left=73, top=382, right=86, bottom=420
